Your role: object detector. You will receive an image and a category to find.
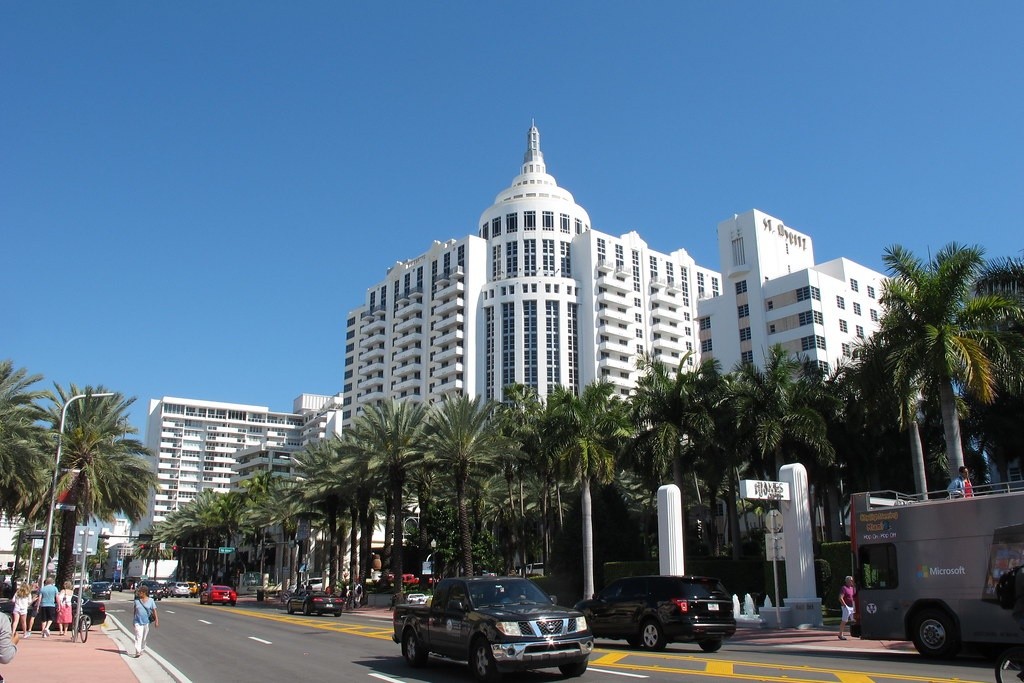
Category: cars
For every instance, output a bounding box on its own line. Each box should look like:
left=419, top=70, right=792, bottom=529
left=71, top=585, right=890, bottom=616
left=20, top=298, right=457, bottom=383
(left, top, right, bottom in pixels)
left=73, top=578, right=90, bottom=590
left=91, top=582, right=111, bottom=600
left=287, top=590, right=344, bottom=617
left=112, top=583, right=122, bottom=592
left=159, top=584, right=169, bottom=598
left=134, top=580, right=163, bottom=601
left=0, top=593, right=106, bottom=631
left=405, top=594, right=427, bottom=604
left=200, top=586, right=236, bottom=606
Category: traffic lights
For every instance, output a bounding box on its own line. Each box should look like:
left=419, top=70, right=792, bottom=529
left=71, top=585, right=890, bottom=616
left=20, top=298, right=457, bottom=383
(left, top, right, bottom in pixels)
left=140, top=544, right=150, bottom=549
left=173, top=546, right=182, bottom=550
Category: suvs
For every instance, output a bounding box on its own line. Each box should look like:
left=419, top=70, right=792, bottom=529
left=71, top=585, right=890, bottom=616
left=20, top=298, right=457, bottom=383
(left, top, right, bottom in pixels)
left=166, top=582, right=191, bottom=598
left=573, top=575, right=737, bottom=653
left=186, top=581, right=197, bottom=598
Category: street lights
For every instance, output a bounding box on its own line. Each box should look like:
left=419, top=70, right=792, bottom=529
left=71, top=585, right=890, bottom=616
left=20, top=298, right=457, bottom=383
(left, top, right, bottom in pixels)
left=36, top=393, right=115, bottom=613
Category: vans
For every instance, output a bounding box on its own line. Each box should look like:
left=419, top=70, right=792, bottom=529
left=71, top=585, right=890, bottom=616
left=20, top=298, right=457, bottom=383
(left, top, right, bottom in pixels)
left=288, top=578, right=324, bottom=593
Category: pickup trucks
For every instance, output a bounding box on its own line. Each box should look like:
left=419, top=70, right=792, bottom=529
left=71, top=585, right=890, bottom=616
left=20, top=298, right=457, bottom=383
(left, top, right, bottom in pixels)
left=392, top=575, right=594, bottom=683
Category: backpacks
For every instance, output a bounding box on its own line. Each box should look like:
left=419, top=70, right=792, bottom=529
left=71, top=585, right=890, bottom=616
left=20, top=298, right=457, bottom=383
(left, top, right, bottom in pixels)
left=996, top=566, right=1024, bottom=610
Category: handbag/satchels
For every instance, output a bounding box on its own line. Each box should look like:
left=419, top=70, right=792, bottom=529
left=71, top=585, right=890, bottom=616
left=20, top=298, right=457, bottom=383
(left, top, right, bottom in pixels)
left=62, top=598, right=68, bottom=608
left=149, top=613, right=156, bottom=623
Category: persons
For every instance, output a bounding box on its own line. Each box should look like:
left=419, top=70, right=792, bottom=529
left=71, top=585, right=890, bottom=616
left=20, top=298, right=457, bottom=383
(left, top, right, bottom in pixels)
left=500, top=584, right=526, bottom=604
left=37, top=578, right=60, bottom=638
left=26, top=580, right=47, bottom=638
left=57, top=581, right=73, bottom=636
left=344, top=585, right=351, bottom=610
left=0, top=612, right=20, bottom=664
left=838, top=576, right=855, bottom=640
left=1015, top=567, right=1024, bottom=630
left=11, top=583, right=39, bottom=639
left=301, top=583, right=312, bottom=590
left=947, top=466, right=973, bottom=497
left=132, top=586, right=159, bottom=658
left=355, top=583, right=363, bottom=608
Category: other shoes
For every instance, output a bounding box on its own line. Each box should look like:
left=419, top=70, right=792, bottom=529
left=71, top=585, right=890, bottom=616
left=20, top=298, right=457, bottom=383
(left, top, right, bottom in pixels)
left=24, top=633, right=29, bottom=638
left=44, top=629, right=50, bottom=636
left=136, top=652, right=142, bottom=657
left=838, top=635, right=846, bottom=640
left=42, top=634, right=46, bottom=638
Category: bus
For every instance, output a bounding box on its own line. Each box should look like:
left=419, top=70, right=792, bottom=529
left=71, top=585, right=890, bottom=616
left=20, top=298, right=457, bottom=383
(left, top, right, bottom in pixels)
left=850, top=478, right=1023, bottom=660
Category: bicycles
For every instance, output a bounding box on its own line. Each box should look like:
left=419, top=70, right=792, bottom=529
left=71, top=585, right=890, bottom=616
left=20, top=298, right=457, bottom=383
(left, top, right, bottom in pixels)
left=994, top=644, right=1024, bottom=683
left=72, top=599, right=90, bottom=643
